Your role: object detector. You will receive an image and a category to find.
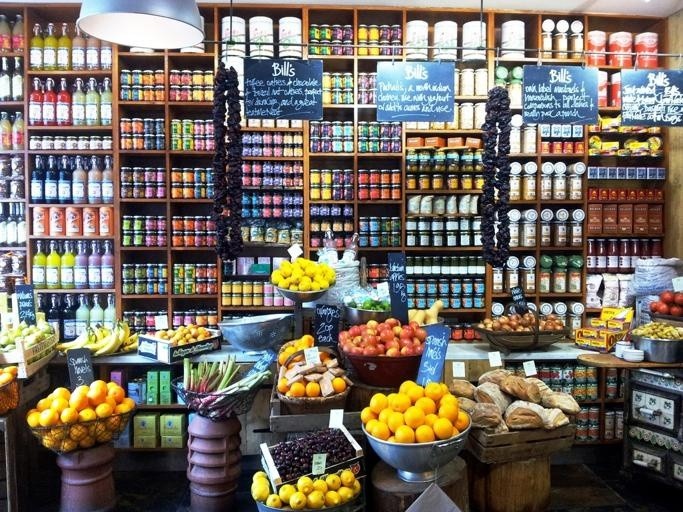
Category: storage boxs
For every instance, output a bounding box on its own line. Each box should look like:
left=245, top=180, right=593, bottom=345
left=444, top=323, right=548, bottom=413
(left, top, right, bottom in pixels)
left=474, top=427, right=578, bottom=463
left=140, top=329, right=222, bottom=365
left=627, top=369, right=683, bottom=481
left=18, top=368, right=52, bottom=405
left=0, top=412, right=31, bottom=512
left=267, top=371, right=364, bottom=455
left=0, top=322, right=61, bottom=376
left=258, top=424, right=362, bottom=492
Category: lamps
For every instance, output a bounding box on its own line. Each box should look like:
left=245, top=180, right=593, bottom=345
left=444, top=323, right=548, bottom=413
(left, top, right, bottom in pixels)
left=76, top=1, right=206, bottom=50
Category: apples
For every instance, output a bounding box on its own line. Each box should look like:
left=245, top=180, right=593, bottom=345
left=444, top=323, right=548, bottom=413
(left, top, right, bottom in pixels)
left=339, top=318, right=426, bottom=356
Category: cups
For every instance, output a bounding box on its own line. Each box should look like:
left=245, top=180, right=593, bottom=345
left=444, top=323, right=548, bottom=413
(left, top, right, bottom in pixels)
left=615, top=341, right=633, bottom=358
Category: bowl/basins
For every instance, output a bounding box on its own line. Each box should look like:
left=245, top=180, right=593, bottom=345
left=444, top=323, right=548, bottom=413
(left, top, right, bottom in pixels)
left=360, top=413, right=472, bottom=483
left=217, top=312, right=295, bottom=357
left=341, top=355, right=421, bottom=388
left=344, top=305, right=390, bottom=327
left=630, top=331, right=683, bottom=363
left=275, top=282, right=328, bottom=306
left=622, top=350, right=644, bottom=362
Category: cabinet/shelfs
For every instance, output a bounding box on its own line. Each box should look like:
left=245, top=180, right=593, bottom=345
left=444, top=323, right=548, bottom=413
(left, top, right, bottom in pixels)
left=490, top=9, right=669, bottom=328
left=67, top=345, right=598, bottom=485
left=0, top=3, right=120, bottom=323
left=118, top=2, right=307, bottom=340
left=305, top=4, right=489, bottom=326
left=575, top=355, right=683, bottom=474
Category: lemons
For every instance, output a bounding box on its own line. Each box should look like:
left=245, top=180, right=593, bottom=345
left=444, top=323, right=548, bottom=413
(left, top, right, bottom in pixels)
left=313, top=479, right=328, bottom=493
left=307, top=491, right=325, bottom=508
left=279, top=484, right=296, bottom=503
left=352, top=480, right=360, bottom=494
left=297, top=476, right=313, bottom=494
left=338, top=487, right=354, bottom=502
left=290, top=491, right=306, bottom=509
left=325, top=491, right=341, bottom=507
left=266, top=494, right=282, bottom=508
left=326, top=474, right=341, bottom=490
left=272, top=257, right=335, bottom=292
left=341, top=470, right=355, bottom=486
left=250, top=471, right=270, bottom=500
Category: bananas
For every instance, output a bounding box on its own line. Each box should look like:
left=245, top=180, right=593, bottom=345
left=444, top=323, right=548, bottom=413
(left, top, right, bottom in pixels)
left=124, top=331, right=143, bottom=350
left=85, top=318, right=129, bottom=356
left=95, top=324, right=111, bottom=340
left=56, top=323, right=96, bottom=357
